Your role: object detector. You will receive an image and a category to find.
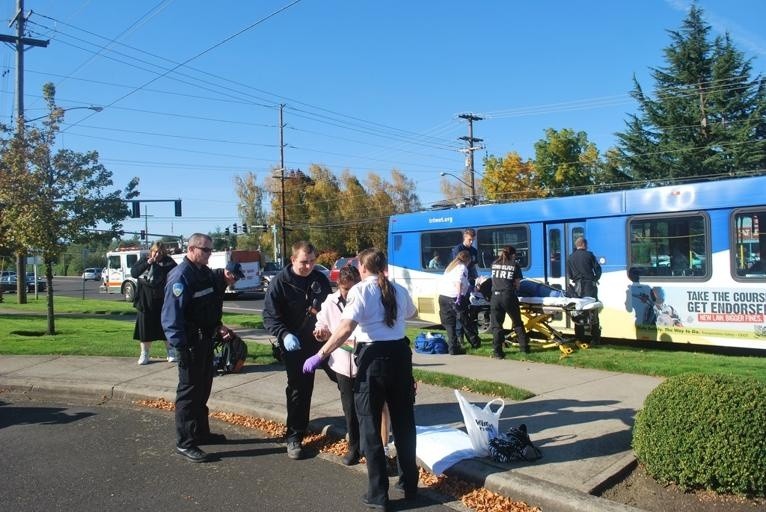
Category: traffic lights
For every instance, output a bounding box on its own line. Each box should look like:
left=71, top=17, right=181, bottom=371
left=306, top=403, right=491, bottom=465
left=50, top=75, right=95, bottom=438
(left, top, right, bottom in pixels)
left=233, top=223, right=237, bottom=233
left=141, top=230, right=145, bottom=240
left=262, top=224, right=267, bottom=232
left=224, top=228, right=229, bottom=236
left=242, top=223, right=247, bottom=233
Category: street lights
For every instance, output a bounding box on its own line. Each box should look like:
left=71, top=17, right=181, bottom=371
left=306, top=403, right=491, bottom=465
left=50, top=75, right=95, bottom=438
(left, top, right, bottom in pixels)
left=440, top=172, right=474, bottom=205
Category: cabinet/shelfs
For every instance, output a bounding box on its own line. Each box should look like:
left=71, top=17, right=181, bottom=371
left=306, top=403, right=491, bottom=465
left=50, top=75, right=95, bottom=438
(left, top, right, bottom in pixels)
left=469, top=291, right=602, bottom=354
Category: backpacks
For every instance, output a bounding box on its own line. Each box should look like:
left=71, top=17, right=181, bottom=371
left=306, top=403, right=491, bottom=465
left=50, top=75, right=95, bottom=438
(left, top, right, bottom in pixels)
left=222, top=334, right=247, bottom=373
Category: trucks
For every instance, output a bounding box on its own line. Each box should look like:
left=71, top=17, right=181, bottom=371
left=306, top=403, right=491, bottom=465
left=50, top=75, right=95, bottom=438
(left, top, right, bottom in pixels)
left=100, top=245, right=262, bottom=305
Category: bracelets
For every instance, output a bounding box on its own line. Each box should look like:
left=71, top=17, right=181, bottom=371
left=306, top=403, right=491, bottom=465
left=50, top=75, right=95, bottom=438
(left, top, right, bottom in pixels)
left=318, top=348, right=327, bottom=358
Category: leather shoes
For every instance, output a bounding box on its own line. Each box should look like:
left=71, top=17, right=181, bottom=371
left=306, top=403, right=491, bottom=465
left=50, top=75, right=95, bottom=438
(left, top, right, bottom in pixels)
left=175, top=446, right=207, bottom=463
left=196, top=434, right=226, bottom=446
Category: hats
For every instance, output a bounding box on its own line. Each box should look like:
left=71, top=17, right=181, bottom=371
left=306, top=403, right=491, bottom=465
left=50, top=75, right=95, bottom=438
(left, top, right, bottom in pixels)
left=226, top=262, right=245, bottom=278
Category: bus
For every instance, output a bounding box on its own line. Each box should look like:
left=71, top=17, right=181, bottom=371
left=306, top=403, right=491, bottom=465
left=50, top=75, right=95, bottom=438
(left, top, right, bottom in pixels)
left=385, top=176, right=765, bottom=351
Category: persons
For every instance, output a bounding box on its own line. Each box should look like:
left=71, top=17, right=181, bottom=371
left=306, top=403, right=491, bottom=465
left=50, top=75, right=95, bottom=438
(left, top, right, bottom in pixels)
left=131, top=240, right=177, bottom=367
left=474, top=274, right=578, bottom=299
left=489, top=245, right=530, bottom=358
left=427, top=250, right=446, bottom=269
left=201, top=262, right=245, bottom=349
left=261, top=239, right=340, bottom=460
left=453, top=227, right=479, bottom=344
left=312, top=265, right=394, bottom=473
left=438, top=250, right=472, bottom=356
left=161, top=232, right=227, bottom=464
left=302, top=246, right=420, bottom=510
left=567, top=236, right=602, bottom=345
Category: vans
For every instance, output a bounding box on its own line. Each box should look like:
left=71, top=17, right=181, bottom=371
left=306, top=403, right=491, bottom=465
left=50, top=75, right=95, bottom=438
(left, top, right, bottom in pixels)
left=0, top=271, right=15, bottom=278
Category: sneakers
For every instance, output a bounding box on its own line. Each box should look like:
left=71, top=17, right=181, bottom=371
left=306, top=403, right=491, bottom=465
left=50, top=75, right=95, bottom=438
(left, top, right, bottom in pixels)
left=394, top=482, right=416, bottom=499
left=167, top=350, right=177, bottom=362
left=287, top=442, right=303, bottom=459
left=360, top=494, right=388, bottom=512
left=343, top=453, right=359, bottom=466
left=386, top=455, right=394, bottom=476
left=138, top=351, right=149, bottom=365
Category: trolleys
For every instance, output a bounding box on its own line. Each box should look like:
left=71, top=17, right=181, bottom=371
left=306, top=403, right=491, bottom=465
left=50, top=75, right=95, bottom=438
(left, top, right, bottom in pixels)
left=464, top=301, right=603, bottom=355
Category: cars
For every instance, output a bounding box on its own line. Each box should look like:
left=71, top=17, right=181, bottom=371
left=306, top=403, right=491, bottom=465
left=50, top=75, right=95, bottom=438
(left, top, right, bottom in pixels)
left=314, top=263, right=329, bottom=277
left=263, top=261, right=284, bottom=293
left=82, top=267, right=101, bottom=281
left=0, top=274, right=47, bottom=292
left=26, top=272, right=41, bottom=280
left=329, top=257, right=388, bottom=285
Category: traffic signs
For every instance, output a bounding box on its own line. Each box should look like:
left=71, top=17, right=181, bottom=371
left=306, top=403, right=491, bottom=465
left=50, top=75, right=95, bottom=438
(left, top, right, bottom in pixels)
left=22, top=106, right=104, bottom=127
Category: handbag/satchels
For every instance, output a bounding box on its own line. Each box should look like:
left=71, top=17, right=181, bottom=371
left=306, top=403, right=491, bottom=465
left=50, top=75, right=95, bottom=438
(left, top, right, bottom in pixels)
left=488, top=424, right=544, bottom=464
left=415, top=333, right=448, bottom=354
left=138, top=262, right=176, bottom=290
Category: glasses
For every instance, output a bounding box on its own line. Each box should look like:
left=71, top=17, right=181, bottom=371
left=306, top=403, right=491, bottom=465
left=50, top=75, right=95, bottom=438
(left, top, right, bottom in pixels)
left=193, top=246, right=212, bottom=253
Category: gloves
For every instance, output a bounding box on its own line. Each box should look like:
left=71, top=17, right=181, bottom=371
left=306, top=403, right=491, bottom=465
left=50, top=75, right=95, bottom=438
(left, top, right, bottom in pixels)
left=284, top=334, right=302, bottom=352
left=303, top=354, right=324, bottom=374
